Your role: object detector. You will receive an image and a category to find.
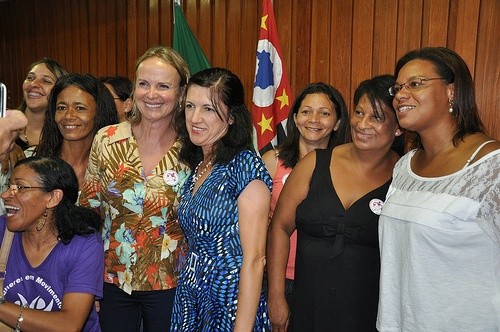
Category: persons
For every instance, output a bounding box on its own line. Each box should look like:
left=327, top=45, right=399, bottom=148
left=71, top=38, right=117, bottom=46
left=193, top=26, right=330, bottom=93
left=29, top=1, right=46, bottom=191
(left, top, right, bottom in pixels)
left=98, top=76, right=134, bottom=123
left=81, top=46, right=191, bottom=332
left=0, top=156, right=104, bottom=332
left=24, top=73, right=119, bottom=206
left=0, top=57, right=69, bottom=216
left=376, top=47, right=500, bottom=331
left=170, top=67, right=272, bottom=332
left=266, top=74, right=405, bottom=332
left=261, top=82, right=350, bottom=306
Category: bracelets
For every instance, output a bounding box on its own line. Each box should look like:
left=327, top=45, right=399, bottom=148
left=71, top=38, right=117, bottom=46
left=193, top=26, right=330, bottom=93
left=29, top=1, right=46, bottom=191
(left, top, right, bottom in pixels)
left=14, top=304, right=30, bottom=332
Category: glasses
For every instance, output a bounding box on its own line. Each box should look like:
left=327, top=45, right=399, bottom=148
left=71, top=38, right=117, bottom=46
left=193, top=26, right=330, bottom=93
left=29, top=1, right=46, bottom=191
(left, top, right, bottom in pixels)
left=2, top=180, right=45, bottom=196
left=388, top=76, right=447, bottom=96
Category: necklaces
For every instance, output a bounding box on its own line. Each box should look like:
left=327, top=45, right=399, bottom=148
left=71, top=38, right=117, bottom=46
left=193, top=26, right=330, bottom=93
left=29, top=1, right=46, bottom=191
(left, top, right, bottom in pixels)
left=24, top=127, right=28, bottom=147
left=190, top=161, right=212, bottom=190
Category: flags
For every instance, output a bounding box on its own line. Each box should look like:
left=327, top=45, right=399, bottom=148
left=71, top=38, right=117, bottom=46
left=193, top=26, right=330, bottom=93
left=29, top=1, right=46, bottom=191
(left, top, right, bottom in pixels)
left=250, top=0, right=294, bottom=159
left=172, top=0, right=211, bottom=76
